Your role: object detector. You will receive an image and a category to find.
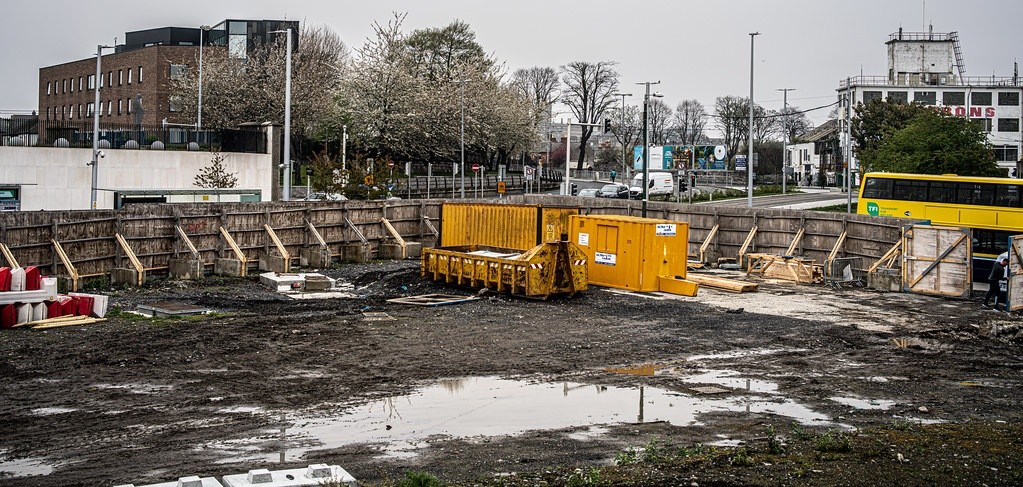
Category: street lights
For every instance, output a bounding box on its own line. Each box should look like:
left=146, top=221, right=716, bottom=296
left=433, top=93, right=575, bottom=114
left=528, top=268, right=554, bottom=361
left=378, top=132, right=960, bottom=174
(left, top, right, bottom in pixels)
left=748, top=31, right=763, bottom=210
left=268, top=29, right=291, bottom=200
left=90, top=45, right=117, bottom=210
left=643, top=93, right=663, bottom=218
left=636, top=80, right=660, bottom=217
left=343, top=125, right=347, bottom=183
left=449, top=79, right=472, bottom=198
left=776, top=89, right=795, bottom=193
left=615, top=94, right=632, bottom=185
left=198, top=25, right=210, bottom=128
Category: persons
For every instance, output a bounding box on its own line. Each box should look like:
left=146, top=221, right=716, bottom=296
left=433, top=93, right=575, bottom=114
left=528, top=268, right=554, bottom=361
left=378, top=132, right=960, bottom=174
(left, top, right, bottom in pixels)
left=982, top=258, right=1009, bottom=312
left=588, top=165, right=593, bottom=173
left=610, top=169, right=616, bottom=183
left=933, top=190, right=943, bottom=201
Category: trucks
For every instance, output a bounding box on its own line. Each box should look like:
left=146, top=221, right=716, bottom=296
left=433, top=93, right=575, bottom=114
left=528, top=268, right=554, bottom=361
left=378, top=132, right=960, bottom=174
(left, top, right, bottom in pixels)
left=632, top=172, right=673, bottom=200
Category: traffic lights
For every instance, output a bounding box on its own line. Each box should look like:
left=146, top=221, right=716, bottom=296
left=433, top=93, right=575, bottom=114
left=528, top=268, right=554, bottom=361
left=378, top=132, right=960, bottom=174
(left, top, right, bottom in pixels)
left=684, top=183, right=687, bottom=191
left=573, top=184, right=578, bottom=196
left=606, top=118, right=611, bottom=131
left=692, top=176, right=695, bottom=187
left=681, top=179, right=685, bottom=191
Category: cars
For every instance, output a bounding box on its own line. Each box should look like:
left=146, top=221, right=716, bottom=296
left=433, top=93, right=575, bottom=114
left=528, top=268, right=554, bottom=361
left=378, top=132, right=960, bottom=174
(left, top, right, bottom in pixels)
left=630, top=187, right=642, bottom=199
left=578, top=189, right=602, bottom=198
left=601, top=185, right=629, bottom=199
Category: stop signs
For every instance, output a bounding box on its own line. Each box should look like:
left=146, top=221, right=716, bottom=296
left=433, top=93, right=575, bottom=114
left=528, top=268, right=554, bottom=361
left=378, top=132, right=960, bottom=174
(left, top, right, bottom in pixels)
left=472, top=164, right=480, bottom=172
left=387, top=160, right=394, bottom=169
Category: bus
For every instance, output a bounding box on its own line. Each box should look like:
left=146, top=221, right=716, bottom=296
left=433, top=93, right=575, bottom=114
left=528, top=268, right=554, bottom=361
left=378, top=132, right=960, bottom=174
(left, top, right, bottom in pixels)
left=856, top=172, right=1023, bottom=284
